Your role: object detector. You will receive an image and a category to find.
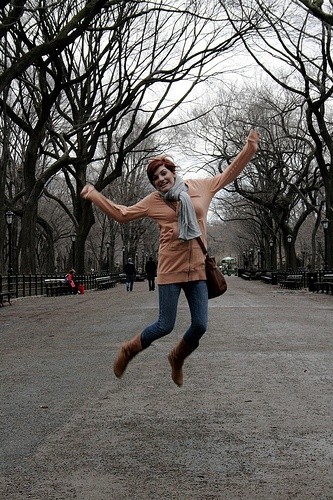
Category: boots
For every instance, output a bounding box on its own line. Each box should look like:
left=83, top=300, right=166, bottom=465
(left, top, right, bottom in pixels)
left=168, top=339, right=199, bottom=388
left=113, top=331, right=142, bottom=379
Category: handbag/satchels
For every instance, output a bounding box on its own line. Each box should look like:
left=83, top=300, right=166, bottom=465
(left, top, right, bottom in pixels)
left=205, top=256, right=228, bottom=299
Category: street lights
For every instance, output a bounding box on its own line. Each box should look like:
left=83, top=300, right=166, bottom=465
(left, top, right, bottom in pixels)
left=106, top=241, right=111, bottom=274
left=6, top=210, right=15, bottom=302
left=243, top=250, right=246, bottom=268
left=257, top=247, right=261, bottom=271
left=122, top=246, right=126, bottom=271
left=70, top=231, right=77, bottom=270
left=249, top=251, right=252, bottom=267
left=270, top=240, right=274, bottom=271
left=141, top=248, right=145, bottom=271
left=287, top=234, right=292, bottom=271
left=321, top=217, right=330, bottom=274
left=136, top=251, right=139, bottom=271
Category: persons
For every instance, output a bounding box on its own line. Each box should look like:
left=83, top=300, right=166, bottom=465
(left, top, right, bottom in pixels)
left=124, top=257, right=136, bottom=292
left=145, top=257, right=157, bottom=291
left=80, top=130, right=260, bottom=388
left=65, top=270, right=81, bottom=295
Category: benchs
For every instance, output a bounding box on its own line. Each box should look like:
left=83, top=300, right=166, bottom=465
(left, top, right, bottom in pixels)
left=261, top=272, right=278, bottom=285
left=0, top=291, right=15, bottom=307
left=119, top=274, right=126, bottom=283
left=96, top=275, right=117, bottom=290
left=136, top=275, right=145, bottom=281
left=46, top=279, right=72, bottom=295
left=314, top=274, right=333, bottom=295
left=242, top=272, right=251, bottom=281
left=281, top=275, right=303, bottom=290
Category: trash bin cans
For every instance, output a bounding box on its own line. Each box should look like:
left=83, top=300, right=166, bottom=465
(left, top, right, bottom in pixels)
left=307, top=273, right=318, bottom=291
left=271, top=273, right=278, bottom=285
